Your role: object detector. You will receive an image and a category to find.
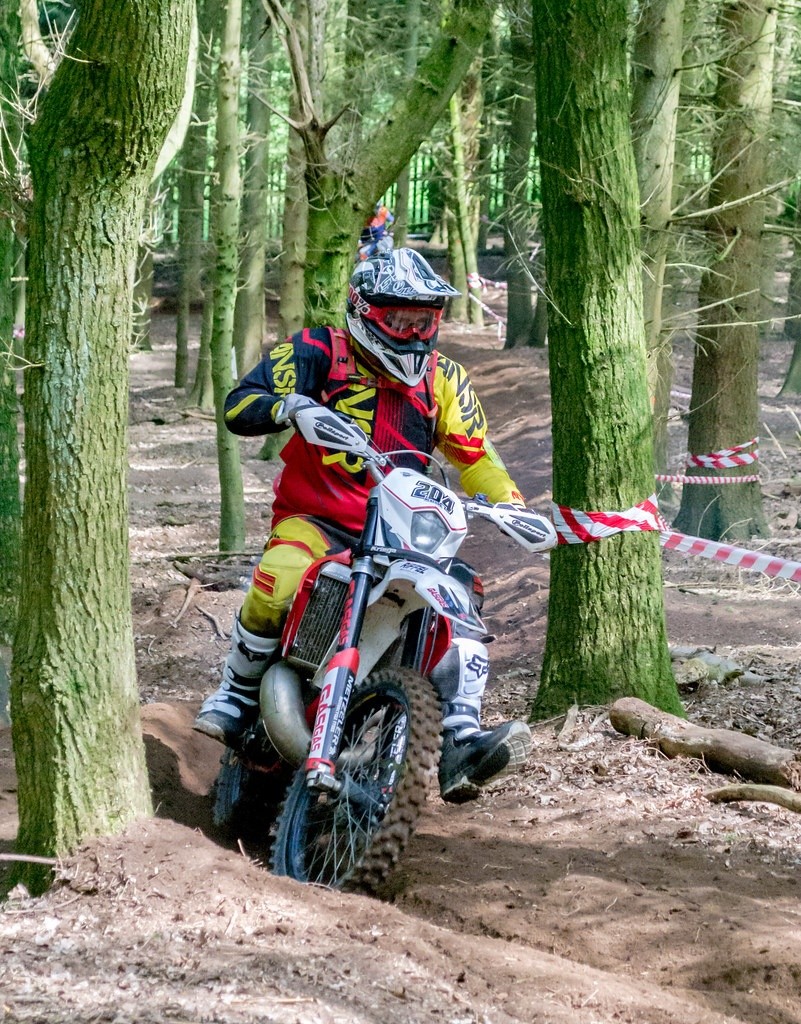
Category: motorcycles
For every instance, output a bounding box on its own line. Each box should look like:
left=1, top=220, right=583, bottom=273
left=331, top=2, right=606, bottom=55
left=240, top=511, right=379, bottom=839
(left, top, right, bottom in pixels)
left=357, top=221, right=397, bottom=265
left=211, top=401, right=559, bottom=901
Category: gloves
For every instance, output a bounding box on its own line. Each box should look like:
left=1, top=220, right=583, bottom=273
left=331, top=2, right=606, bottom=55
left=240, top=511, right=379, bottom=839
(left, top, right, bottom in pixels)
left=276, top=393, right=319, bottom=423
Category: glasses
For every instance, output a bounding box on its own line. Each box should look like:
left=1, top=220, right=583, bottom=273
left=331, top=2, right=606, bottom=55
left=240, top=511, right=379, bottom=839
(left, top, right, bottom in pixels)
left=348, top=282, right=445, bottom=341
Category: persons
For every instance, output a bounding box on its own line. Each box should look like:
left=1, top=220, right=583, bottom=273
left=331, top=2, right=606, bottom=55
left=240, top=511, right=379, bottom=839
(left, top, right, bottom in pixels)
left=353, top=204, right=395, bottom=262
left=191, top=248, right=532, bottom=805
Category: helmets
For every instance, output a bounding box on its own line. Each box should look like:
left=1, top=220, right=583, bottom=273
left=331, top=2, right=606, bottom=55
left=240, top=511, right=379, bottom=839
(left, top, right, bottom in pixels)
left=347, top=248, right=463, bottom=387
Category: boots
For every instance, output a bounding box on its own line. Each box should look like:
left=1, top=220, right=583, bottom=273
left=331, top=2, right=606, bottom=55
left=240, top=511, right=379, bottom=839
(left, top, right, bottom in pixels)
left=431, top=637, right=533, bottom=804
left=192, top=616, right=282, bottom=739
